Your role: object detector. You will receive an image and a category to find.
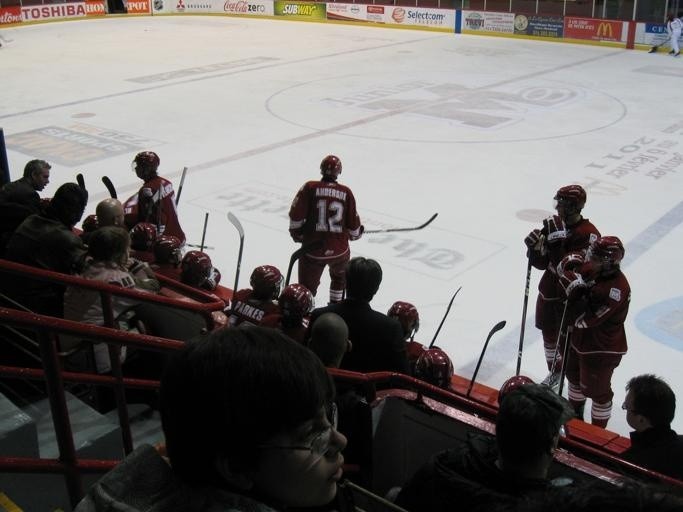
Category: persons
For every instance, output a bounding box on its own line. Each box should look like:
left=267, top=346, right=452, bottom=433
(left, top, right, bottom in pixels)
left=666, top=12, right=683, bottom=56
left=1, top=151, right=681, bottom=511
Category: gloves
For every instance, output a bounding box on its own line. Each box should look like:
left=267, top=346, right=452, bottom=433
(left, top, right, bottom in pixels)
left=561, top=254, right=584, bottom=274
left=349, top=226, right=364, bottom=241
left=525, top=230, right=545, bottom=258
left=291, top=234, right=304, bottom=243
left=559, top=269, right=585, bottom=298
left=543, top=217, right=567, bottom=241
left=210, top=268, right=221, bottom=290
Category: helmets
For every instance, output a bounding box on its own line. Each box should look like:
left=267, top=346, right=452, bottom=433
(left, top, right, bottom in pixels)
left=181, top=251, right=212, bottom=282
left=130, top=152, right=159, bottom=176
left=555, top=185, right=586, bottom=220
left=130, top=223, right=157, bottom=251
left=499, top=376, right=536, bottom=404
left=82, top=215, right=101, bottom=231
left=321, top=156, right=342, bottom=178
left=388, top=302, right=419, bottom=340
left=278, top=284, right=313, bottom=321
left=415, top=348, right=453, bottom=388
left=250, top=265, right=283, bottom=298
left=153, top=234, right=182, bottom=267
left=586, top=237, right=625, bottom=271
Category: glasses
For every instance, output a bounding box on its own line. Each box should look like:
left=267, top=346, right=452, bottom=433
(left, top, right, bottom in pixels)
left=279, top=403, right=338, bottom=466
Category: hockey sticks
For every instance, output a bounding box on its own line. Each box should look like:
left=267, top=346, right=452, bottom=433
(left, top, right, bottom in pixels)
left=363, top=213, right=437, bottom=234
left=429, top=287, right=463, bottom=348
left=227, top=212, right=245, bottom=306
left=285, top=241, right=321, bottom=287
left=100, top=175, right=119, bottom=199
left=466, top=320, right=505, bottom=395
